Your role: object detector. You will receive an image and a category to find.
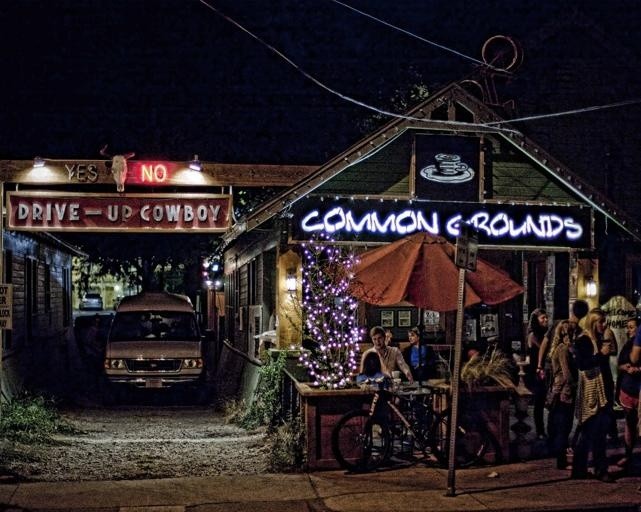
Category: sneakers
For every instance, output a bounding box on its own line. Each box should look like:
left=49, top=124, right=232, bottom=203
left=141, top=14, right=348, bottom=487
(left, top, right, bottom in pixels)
left=617, top=454, right=635, bottom=467
left=595, top=469, right=613, bottom=482
left=571, top=471, right=592, bottom=478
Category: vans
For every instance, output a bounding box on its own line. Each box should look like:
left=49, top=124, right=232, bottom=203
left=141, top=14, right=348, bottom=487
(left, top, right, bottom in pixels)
left=101, top=289, right=203, bottom=395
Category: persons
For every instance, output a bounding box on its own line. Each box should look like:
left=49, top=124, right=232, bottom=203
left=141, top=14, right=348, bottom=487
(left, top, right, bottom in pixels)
left=527, top=299, right=641, bottom=483
left=360, top=325, right=440, bottom=388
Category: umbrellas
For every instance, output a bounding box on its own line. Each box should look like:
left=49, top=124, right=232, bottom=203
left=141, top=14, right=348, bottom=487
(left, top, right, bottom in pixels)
left=319, top=229, right=527, bottom=391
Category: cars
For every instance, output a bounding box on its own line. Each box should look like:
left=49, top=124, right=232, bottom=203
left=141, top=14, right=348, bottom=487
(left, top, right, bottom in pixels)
left=78, top=293, right=103, bottom=310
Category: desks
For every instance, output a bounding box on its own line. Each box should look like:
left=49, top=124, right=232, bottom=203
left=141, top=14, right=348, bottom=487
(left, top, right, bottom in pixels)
left=394, top=385, right=431, bottom=442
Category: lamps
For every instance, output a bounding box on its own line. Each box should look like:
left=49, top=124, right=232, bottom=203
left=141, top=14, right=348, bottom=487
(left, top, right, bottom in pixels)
left=285, top=266, right=298, bottom=291
left=33, top=157, right=44, bottom=166
left=584, top=273, right=598, bottom=297
left=188, top=155, right=202, bottom=172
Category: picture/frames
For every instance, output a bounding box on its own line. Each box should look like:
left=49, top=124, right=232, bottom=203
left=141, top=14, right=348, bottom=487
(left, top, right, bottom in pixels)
left=380, top=310, right=395, bottom=329
left=397, top=310, right=412, bottom=327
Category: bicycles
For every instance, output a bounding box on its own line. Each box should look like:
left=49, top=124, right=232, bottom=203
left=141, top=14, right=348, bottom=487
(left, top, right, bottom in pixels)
left=331, top=375, right=490, bottom=473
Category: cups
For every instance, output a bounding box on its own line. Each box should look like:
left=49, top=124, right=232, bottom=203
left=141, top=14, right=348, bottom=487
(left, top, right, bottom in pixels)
left=392, top=370, right=400, bottom=379
